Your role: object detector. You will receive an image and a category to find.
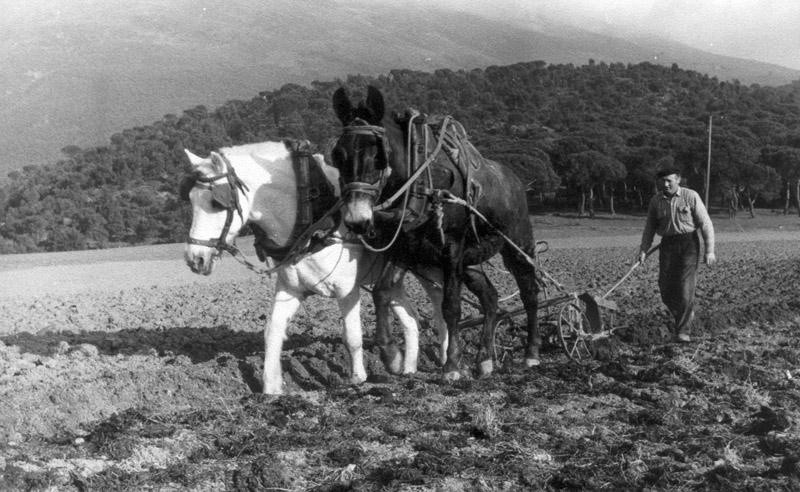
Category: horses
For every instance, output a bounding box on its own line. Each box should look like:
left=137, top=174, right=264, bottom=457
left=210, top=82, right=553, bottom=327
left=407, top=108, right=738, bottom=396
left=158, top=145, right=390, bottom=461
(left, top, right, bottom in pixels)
left=183, top=140, right=449, bottom=400
left=333, top=85, right=540, bottom=380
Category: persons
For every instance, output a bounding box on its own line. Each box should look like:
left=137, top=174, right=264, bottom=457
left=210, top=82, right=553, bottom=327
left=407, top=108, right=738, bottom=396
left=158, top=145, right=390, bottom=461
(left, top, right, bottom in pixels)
left=635, top=166, right=717, bottom=342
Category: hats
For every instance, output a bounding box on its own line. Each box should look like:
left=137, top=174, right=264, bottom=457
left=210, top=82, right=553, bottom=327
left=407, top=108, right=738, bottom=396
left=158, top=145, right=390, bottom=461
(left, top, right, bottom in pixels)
left=658, top=169, right=679, bottom=178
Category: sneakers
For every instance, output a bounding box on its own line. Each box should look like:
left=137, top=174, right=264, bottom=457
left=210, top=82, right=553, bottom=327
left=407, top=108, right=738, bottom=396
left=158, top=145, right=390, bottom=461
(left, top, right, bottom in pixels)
left=675, top=333, right=691, bottom=343
